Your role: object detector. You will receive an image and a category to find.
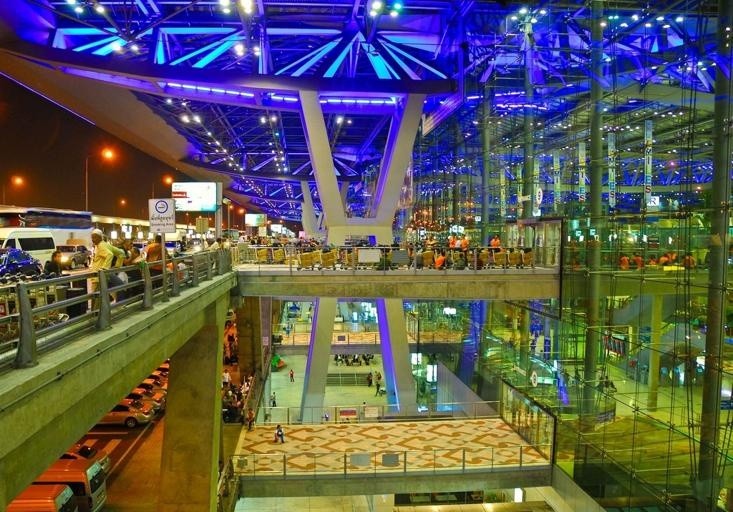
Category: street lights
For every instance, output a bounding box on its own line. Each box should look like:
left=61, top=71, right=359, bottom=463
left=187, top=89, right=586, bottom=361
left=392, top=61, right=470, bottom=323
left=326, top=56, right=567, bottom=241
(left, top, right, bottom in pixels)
left=229, top=204, right=247, bottom=230
left=2, top=174, right=27, bottom=205
left=84, top=145, right=116, bottom=212
left=149, top=172, right=174, bottom=198
left=109, top=197, right=127, bottom=216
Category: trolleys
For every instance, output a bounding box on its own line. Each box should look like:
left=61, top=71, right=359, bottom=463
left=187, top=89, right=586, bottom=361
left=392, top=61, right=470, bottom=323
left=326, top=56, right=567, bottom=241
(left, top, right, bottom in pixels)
left=253, top=243, right=532, bottom=271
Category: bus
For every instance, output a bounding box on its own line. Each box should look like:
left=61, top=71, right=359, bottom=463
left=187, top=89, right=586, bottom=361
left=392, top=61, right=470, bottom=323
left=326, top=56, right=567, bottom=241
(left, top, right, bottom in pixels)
left=1, top=205, right=93, bottom=259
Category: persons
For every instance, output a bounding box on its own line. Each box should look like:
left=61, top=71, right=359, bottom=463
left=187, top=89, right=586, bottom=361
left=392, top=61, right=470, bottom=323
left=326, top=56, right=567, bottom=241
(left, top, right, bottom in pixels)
left=489, top=234, right=500, bottom=252
left=210, top=238, right=222, bottom=251
left=215, top=319, right=384, bottom=485
left=88, top=229, right=125, bottom=312
left=237, top=233, right=319, bottom=247
left=391, top=241, right=400, bottom=250
left=45, top=251, right=63, bottom=277
left=113, top=234, right=189, bottom=299
left=471, top=253, right=483, bottom=269
left=408, top=233, right=468, bottom=268
left=610, top=248, right=697, bottom=273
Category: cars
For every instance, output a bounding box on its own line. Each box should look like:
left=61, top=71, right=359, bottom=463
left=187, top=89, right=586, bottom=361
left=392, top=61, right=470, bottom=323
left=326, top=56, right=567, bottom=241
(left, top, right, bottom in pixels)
left=628, top=341, right=704, bottom=387
left=54, top=244, right=93, bottom=271
left=129, top=229, right=210, bottom=257
left=61, top=445, right=113, bottom=475
left=224, top=308, right=234, bottom=326
left=96, top=358, right=169, bottom=428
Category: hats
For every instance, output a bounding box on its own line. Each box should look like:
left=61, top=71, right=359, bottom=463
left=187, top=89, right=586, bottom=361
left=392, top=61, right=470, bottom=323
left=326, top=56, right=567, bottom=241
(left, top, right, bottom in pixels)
left=92, top=229, right=103, bottom=238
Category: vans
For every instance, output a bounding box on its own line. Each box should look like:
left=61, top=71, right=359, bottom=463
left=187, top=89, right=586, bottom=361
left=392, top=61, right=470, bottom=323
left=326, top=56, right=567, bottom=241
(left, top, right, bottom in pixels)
left=0, top=225, right=56, bottom=269
left=32, top=458, right=108, bottom=512
left=4, top=484, right=80, bottom=512
